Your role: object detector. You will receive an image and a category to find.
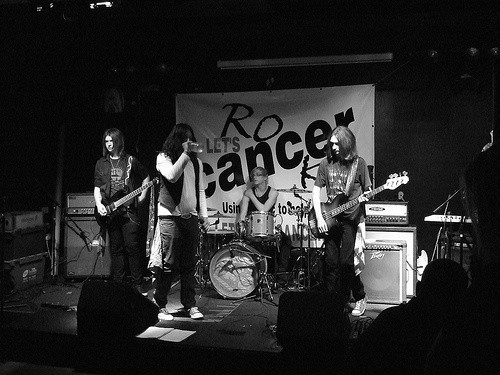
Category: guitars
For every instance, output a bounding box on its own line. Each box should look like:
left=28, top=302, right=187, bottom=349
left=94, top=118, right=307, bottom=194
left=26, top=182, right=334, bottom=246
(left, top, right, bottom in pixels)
left=92, top=177, right=161, bottom=230
left=306, top=169, right=410, bottom=241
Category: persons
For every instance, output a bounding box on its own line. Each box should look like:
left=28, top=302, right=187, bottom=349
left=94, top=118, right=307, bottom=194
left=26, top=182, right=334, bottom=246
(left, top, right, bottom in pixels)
left=93, top=128, right=150, bottom=297
left=240, top=167, right=296, bottom=291
left=155, top=123, right=210, bottom=321
left=312, top=126, right=372, bottom=316
left=352, top=259, right=468, bottom=375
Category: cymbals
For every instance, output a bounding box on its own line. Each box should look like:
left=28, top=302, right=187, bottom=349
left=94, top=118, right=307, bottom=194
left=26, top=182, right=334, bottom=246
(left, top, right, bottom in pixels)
left=206, top=208, right=220, bottom=211
left=274, top=187, right=311, bottom=193
left=206, top=214, right=238, bottom=219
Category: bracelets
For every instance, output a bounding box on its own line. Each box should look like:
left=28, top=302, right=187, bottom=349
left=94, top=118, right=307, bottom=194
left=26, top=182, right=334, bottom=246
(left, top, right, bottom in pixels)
left=185, top=150, right=190, bottom=153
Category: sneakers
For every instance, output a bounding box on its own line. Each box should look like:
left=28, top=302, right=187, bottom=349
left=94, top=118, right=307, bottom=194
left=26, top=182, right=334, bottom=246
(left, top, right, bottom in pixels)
left=352, top=294, right=367, bottom=316
left=189, top=307, right=203, bottom=318
left=158, top=308, right=173, bottom=321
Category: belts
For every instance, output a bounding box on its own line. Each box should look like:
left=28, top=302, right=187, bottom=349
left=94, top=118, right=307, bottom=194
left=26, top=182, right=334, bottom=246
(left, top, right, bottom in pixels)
left=159, top=213, right=198, bottom=220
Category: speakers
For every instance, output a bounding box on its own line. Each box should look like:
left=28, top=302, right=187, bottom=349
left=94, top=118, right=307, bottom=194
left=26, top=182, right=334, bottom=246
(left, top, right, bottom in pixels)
left=365, top=226, right=418, bottom=295
left=276, top=291, right=351, bottom=346
left=76, top=279, right=160, bottom=338
left=64, top=217, right=112, bottom=280
left=359, top=243, right=407, bottom=305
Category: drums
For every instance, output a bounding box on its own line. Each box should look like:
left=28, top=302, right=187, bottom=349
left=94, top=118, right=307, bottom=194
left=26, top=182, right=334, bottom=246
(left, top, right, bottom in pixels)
left=208, top=244, right=271, bottom=300
left=246, top=210, right=276, bottom=242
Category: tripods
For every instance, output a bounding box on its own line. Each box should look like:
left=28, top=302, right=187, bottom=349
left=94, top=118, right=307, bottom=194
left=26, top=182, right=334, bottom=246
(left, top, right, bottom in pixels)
left=283, top=195, right=320, bottom=291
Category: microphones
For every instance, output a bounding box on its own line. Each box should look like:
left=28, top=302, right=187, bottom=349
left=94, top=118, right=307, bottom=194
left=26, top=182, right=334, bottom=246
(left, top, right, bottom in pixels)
left=228, top=246, right=234, bottom=258
